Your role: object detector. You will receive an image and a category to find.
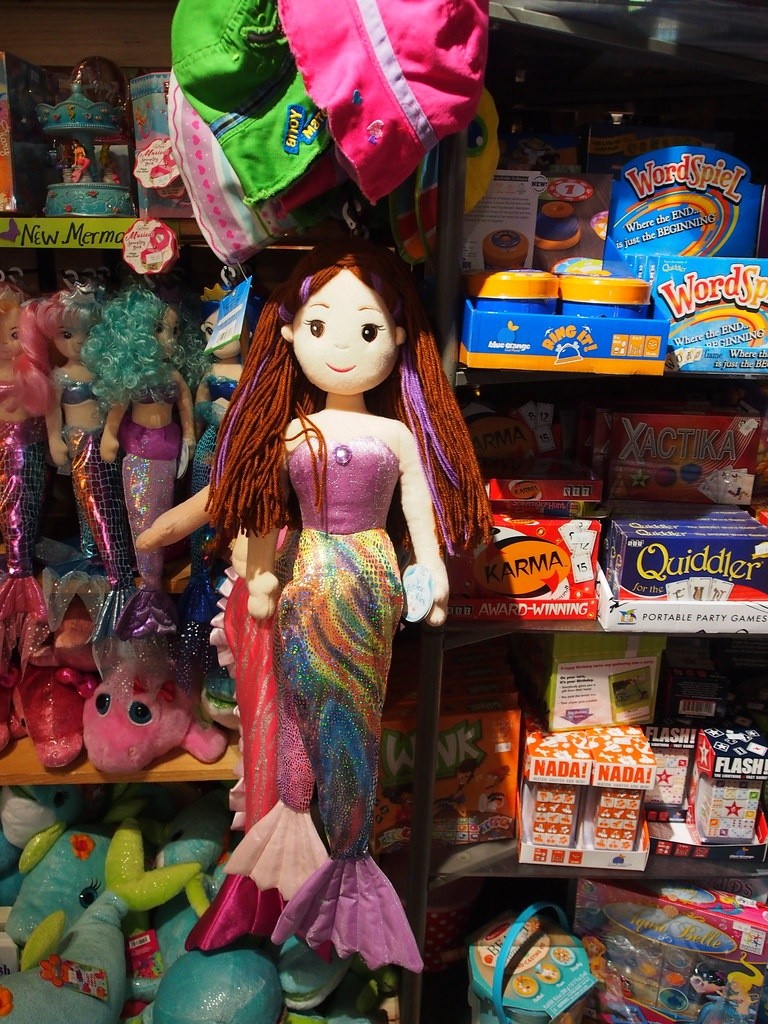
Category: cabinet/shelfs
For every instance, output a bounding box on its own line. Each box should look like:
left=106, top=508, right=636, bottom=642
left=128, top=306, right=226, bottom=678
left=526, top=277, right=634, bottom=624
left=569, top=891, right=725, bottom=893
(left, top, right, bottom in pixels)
left=374, top=69, right=768, bottom=1024
left=0, top=214, right=313, bottom=793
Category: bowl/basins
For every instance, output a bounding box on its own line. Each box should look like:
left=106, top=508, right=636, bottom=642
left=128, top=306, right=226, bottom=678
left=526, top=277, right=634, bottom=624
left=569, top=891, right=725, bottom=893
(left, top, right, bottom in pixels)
left=534, top=201, right=581, bottom=251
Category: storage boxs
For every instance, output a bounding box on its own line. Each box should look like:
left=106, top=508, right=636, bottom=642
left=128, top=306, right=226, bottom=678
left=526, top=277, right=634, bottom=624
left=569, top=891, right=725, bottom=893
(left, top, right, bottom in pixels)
left=572, top=873, right=768, bottom=1024
left=458, top=290, right=670, bottom=377
left=459, top=399, right=768, bottom=633
left=348, top=624, right=768, bottom=872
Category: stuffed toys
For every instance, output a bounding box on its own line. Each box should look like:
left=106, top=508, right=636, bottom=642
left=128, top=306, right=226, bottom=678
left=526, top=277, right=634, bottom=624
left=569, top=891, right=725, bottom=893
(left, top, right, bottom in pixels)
left=0, top=267, right=245, bottom=776
left=136, top=235, right=492, bottom=972
left=0, top=780, right=402, bottom=1024
